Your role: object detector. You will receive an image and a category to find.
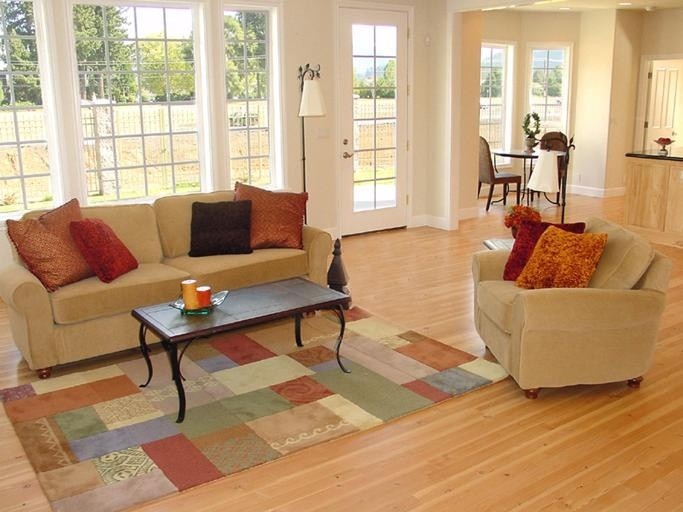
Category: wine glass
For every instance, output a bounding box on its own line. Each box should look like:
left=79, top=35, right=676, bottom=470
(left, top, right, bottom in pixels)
left=524, top=137, right=534, bottom=153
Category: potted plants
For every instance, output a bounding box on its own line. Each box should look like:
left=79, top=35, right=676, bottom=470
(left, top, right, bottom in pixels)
left=522, top=112, right=541, bottom=154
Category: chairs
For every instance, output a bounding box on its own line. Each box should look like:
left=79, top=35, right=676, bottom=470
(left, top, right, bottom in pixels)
left=471, top=215, right=673, bottom=400
left=479, top=137, right=521, bottom=211
left=540, top=132, right=569, bottom=204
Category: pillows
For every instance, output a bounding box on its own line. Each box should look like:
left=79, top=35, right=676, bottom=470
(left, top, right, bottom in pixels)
left=515, top=225, right=608, bottom=289
left=579, top=215, right=656, bottom=291
left=503, top=221, right=586, bottom=282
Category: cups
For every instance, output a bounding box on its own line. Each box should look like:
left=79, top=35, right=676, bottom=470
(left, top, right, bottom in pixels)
left=181, top=279, right=197, bottom=308
left=196, top=286, right=209, bottom=306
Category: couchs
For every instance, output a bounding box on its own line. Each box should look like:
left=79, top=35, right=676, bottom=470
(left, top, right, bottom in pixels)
left=0, top=181, right=333, bottom=379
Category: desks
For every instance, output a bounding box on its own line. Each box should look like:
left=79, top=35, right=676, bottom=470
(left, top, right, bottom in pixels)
left=490, top=149, right=568, bottom=208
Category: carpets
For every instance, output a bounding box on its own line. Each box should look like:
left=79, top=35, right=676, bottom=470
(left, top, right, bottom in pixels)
left=1, top=305, right=511, bottom=512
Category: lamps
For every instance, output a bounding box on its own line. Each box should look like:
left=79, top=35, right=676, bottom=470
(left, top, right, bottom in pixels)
left=526, top=133, right=575, bottom=225
left=298, top=62, right=325, bottom=226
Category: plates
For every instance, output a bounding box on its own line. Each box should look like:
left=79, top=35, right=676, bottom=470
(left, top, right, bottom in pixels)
left=168, top=290, right=228, bottom=314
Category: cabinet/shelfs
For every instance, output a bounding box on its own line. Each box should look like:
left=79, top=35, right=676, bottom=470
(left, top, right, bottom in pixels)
left=624, top=156, right=683, bottom=249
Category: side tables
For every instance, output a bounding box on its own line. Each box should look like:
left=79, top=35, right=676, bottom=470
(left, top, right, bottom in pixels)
left=482, top=238, right=515, bottom=253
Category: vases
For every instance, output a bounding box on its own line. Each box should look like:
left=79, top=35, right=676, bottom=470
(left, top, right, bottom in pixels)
left=510, top=225, right=518, bottom=239
left=659, top=149, right=668, bottom=157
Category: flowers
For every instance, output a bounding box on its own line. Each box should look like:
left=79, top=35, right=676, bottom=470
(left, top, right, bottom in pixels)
left=654, top=137, right=675, bottom=150
left=503, top=203, right=541, bottom=229
